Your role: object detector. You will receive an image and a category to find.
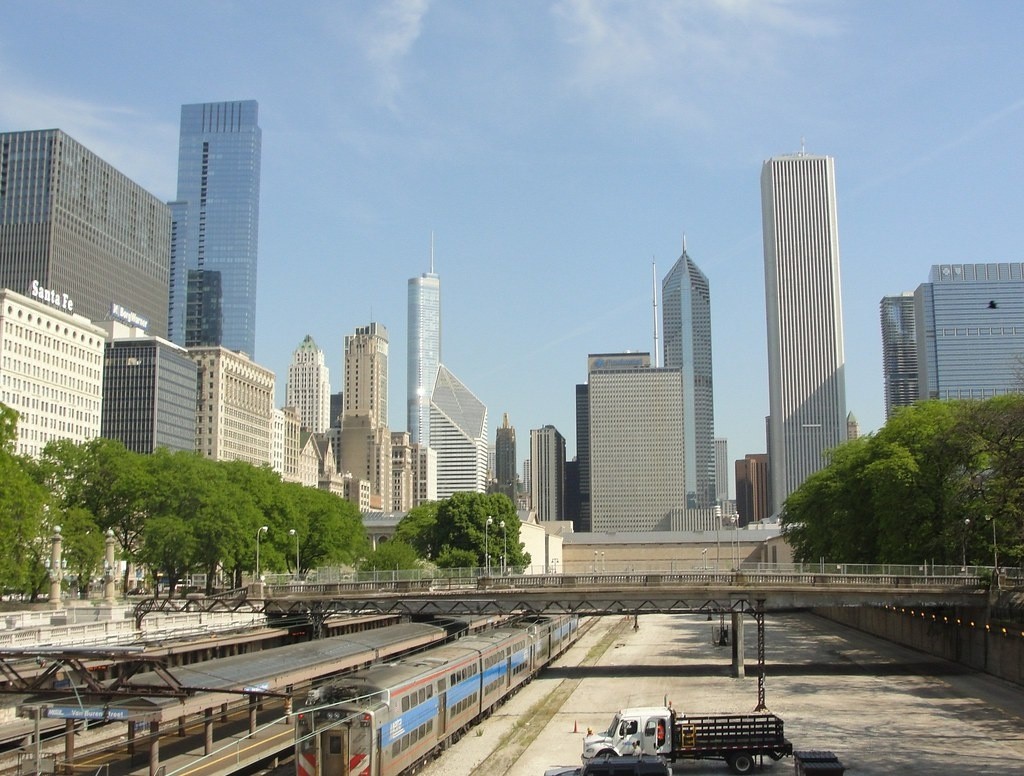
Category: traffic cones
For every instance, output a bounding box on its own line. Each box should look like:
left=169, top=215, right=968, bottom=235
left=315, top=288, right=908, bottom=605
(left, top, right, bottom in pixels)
left=573, top=721, right=577, bottom=733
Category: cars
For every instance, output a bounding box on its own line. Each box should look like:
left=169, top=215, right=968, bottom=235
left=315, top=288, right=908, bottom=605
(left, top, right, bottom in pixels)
left=126, top=587, right=150, bottom=595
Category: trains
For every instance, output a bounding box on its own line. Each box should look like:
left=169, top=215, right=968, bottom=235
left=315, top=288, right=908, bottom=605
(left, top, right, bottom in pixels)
left=0, top=611, right=578, bottom=775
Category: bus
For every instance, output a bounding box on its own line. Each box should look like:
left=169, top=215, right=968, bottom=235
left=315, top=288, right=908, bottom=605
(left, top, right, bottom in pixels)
left=163, top=579, right=192, bottom=590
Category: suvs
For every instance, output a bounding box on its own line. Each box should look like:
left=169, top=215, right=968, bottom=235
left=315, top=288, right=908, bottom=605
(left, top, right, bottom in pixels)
left=544, top=755, right=672, bottom=776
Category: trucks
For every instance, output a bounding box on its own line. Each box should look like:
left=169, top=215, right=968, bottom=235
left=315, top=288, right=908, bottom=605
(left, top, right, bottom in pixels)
left=580, top=694, right=793, bottom=774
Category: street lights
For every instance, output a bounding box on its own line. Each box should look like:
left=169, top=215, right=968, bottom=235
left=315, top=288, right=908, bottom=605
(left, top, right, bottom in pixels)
left=291, top=529, right=301, bottom=581
left=701, top=549, right=707, bottom=571
left=594, top=551, right=598, bottom=574
left=731, top=514, right=741, bottom=574
left=256, top=526, right=268, bottom=581
left=485, top=518, right=494, bottom=577
left=500, top=520, right=506, bottom=577
left=602, top=551, right=605, bottom=573
